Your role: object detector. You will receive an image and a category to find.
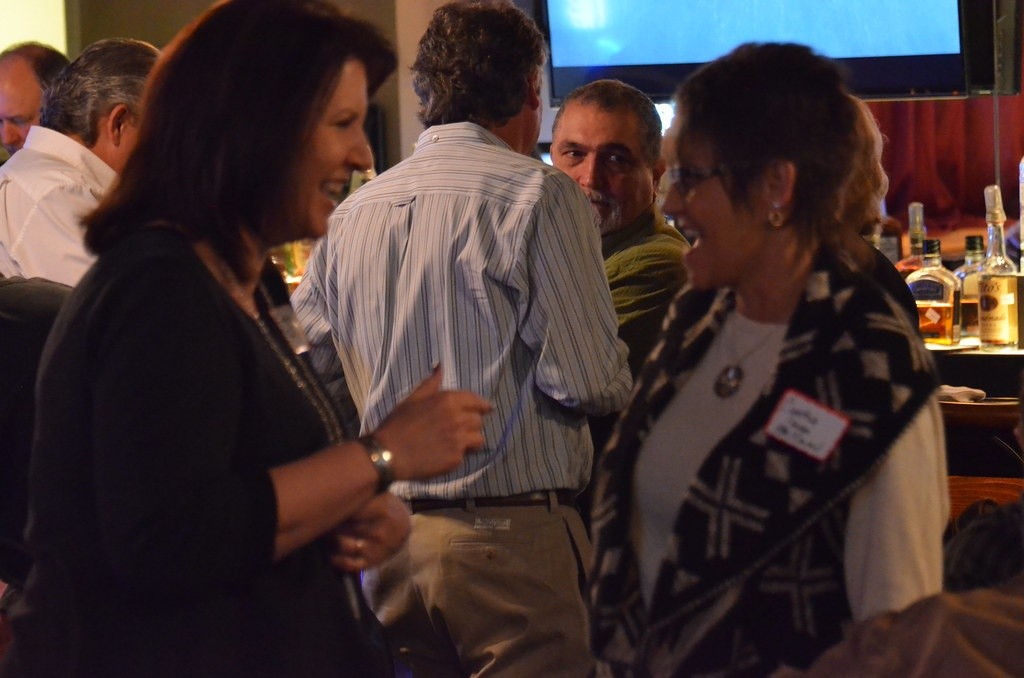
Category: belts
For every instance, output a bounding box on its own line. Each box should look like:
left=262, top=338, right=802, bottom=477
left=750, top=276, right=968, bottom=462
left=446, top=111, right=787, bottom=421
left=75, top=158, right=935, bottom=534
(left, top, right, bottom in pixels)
left=402, top=488, right=583, bottom=515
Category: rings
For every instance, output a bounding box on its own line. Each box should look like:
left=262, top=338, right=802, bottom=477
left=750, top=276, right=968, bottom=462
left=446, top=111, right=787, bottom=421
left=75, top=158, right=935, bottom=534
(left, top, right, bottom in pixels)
left=353, top=536, right=364, bottom=558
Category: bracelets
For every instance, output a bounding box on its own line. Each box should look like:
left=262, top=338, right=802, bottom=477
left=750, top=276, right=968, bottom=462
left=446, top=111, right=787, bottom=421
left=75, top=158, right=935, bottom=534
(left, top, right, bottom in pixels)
left=358, top=431, right=395, bottom=493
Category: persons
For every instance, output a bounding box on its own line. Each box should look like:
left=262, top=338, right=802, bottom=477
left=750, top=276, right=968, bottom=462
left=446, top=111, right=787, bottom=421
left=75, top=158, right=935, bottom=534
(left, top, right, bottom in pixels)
left=289, top=0, right=633, bottom=678
left=585, top=43, right=948, bottom=678
left=836, top=101, right=918, bottom=329
left=770, top=502, right=1024, bottom=678
left=0, top=0, right=495, bottom=678
left=548, top=81, right=692, bottom=538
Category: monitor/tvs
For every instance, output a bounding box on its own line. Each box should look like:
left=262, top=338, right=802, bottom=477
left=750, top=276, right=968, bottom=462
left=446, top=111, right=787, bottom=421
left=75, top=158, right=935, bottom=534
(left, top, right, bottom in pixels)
left=537, top=0, right=970, bottom=108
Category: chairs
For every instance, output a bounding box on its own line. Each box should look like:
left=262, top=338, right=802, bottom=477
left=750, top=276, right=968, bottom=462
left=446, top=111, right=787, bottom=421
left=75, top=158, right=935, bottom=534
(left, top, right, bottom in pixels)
left=936, top=400, right=1024, bottom=534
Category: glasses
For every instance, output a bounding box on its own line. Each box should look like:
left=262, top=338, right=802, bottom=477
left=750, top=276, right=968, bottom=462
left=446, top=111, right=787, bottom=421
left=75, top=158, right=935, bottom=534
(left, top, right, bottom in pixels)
left=652, top=161, right=731, bottom=198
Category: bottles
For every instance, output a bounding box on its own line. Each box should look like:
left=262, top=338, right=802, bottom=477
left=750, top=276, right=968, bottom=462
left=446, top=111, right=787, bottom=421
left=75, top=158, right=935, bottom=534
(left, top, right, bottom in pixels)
left=977, top=185, right=1020, bottom=349
left=889, top=202, right=943, bottom=282
left=950, top=235, right=991, bottom=339
left=903, top=238, right=962, bottom=346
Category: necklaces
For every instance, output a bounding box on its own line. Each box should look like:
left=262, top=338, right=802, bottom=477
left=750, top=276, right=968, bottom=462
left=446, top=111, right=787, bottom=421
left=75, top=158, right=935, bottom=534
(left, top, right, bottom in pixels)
left=713, top=306, right=788, bottom=400
left=222, top=264, right=342, bottom=445
